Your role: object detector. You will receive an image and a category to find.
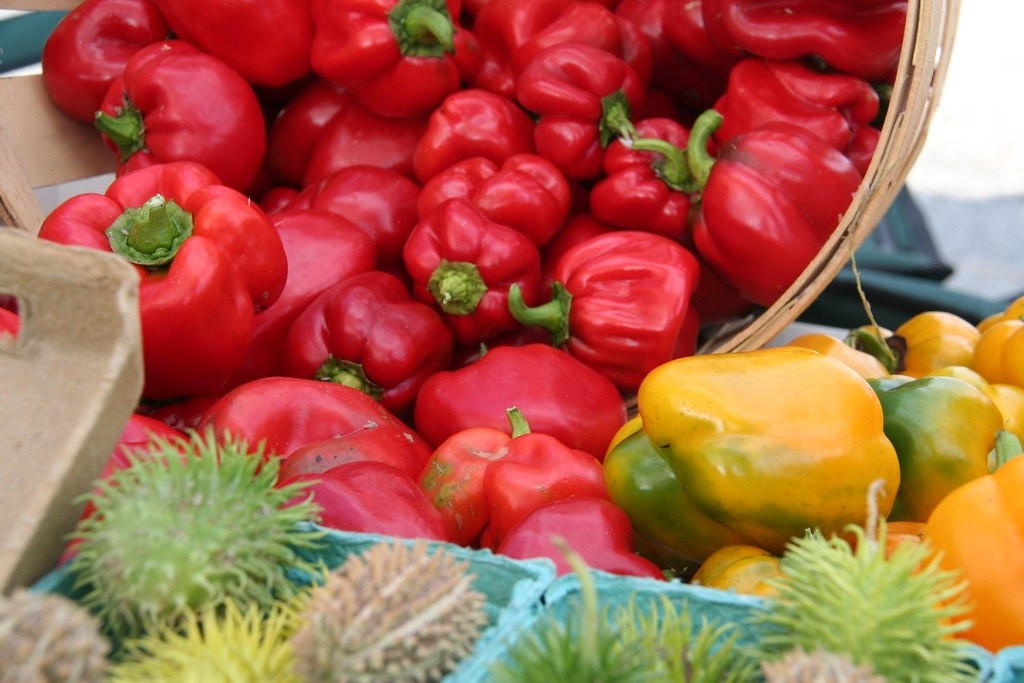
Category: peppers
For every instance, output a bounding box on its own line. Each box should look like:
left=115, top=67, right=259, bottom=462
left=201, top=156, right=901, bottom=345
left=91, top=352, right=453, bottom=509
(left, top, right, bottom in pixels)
left=0, top=0, right=1024, bottom=659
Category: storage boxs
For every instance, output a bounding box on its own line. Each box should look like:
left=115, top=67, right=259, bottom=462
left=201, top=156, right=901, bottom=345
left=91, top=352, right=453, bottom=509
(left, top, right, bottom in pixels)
left=0, top=224, right=1024, bottom=682
